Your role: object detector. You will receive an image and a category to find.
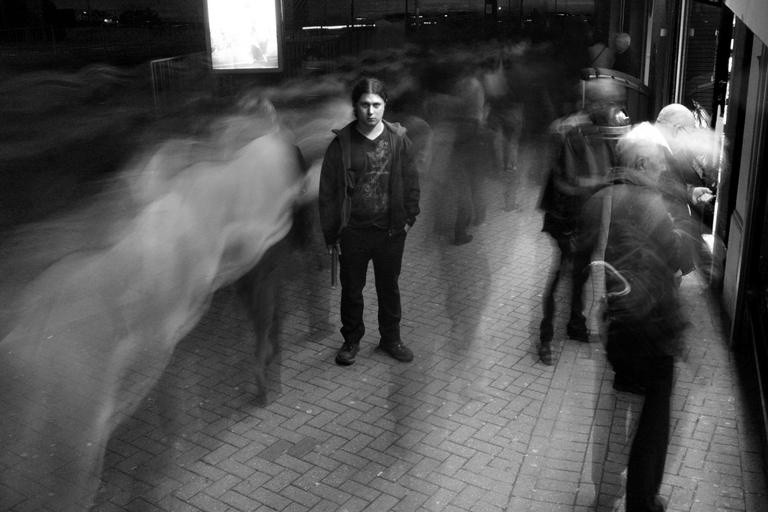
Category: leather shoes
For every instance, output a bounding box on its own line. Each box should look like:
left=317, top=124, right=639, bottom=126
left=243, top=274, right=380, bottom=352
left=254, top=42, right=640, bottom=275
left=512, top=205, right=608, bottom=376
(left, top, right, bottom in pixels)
left=566, top=328, right=600, bottom=342
left=379, top=338, right=414, bottom=362
left=538, top=341, right=553, bottom=366
left=335, top=339, right=359, bottom=367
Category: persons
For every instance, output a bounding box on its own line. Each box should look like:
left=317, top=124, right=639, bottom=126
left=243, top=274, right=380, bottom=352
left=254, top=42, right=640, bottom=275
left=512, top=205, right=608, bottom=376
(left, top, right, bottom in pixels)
left=224, top=97, right=315, bottom=405
left=531, top=80, right=634, bottom=368
left=569, top=99, right=724, bottom=511
left=388, top=31, right=639, bottom=246
left=317, top=77, right=421, bottom=366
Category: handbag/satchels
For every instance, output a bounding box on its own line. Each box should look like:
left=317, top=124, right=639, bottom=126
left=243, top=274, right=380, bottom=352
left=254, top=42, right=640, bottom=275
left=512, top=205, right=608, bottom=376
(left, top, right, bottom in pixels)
left=579, top=186, right=633, bottom=337
